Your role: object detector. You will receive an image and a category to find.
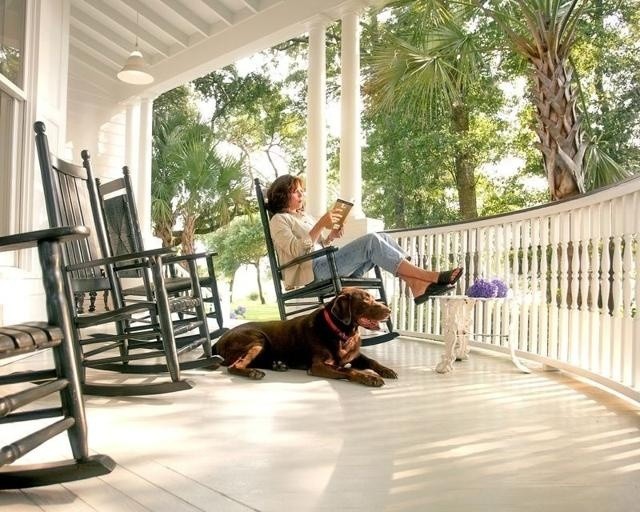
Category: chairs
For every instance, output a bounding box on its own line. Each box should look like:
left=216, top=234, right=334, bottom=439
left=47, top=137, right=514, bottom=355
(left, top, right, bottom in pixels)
left=254, top=177, right=400, bottom=347
left=33, top=120, right=225, bottom=397
left=94, top=166, right=231, bottom=367
left=0, top=225, right=117, bottom=490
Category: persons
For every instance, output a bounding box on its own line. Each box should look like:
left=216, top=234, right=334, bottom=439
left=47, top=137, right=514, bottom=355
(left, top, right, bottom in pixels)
left=265, top=174, right=464, bottom=305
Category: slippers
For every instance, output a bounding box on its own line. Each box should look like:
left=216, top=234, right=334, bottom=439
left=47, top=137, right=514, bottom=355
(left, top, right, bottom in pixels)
left=438, top=267, right=463, bottom=284
left=415, top=283, right=455, bottom=305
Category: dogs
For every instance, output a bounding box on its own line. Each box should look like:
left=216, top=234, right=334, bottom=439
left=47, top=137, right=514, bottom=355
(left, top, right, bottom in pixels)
left=197, top=286, right=399, bottom=387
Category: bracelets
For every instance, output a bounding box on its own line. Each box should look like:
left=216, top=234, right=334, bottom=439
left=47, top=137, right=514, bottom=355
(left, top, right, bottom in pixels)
left=323, top=235, right=334, bottom=245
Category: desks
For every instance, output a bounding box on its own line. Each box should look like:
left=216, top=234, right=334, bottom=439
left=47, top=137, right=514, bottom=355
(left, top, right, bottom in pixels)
left=428, top=290, right=533, bottom=374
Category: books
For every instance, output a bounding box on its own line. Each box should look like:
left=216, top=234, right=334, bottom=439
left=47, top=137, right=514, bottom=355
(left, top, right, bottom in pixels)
left=326, top=197, right=355, bottom=231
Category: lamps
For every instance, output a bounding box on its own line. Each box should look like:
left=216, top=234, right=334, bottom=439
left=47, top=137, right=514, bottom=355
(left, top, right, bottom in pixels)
left=116, top=8, right=155, bottom=85
